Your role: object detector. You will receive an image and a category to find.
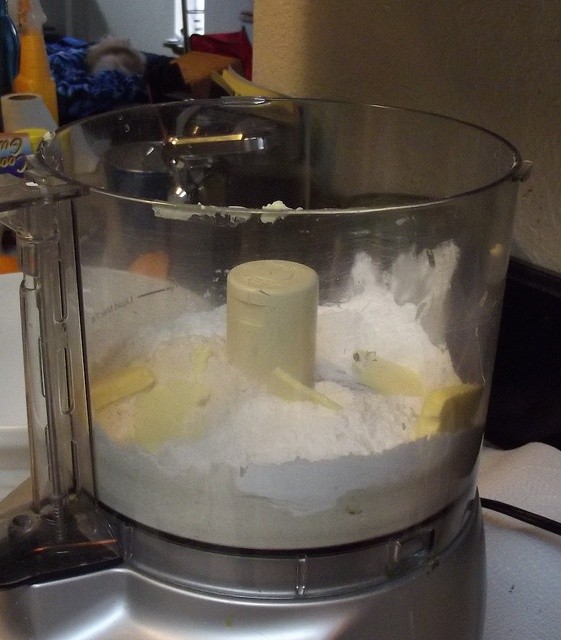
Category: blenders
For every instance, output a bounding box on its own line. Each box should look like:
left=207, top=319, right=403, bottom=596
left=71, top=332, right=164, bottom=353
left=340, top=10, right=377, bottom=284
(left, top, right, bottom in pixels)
left=0, top=99, right=533, bottom=639
